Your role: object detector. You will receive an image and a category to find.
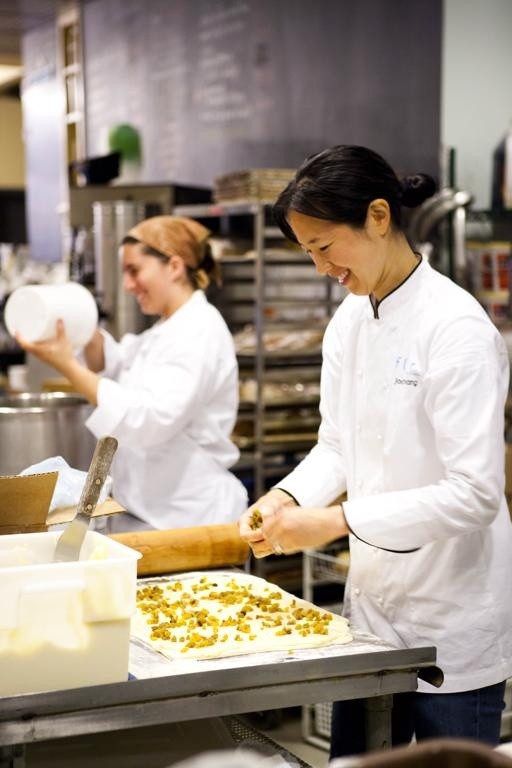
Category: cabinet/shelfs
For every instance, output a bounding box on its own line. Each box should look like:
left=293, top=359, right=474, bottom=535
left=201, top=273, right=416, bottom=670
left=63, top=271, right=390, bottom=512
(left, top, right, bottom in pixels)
left=174, top=203, right=349, bottom=591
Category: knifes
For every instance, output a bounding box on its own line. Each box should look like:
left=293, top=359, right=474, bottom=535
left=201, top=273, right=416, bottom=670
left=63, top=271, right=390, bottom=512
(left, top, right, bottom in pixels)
left=52, top=437, right=120, bottom=562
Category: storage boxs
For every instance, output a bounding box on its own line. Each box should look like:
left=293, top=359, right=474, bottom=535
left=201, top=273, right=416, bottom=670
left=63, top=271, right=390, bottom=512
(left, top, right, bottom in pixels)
left=0, top=529, right=143, bottom=701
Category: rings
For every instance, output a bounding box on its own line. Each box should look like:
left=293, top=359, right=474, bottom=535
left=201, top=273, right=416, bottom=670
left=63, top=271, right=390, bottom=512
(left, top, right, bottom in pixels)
left=272, top=543, right=285, bottom=555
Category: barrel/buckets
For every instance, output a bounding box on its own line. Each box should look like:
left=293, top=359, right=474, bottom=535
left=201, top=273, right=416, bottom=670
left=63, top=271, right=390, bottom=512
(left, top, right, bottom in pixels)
left=4, top=280, right=97, bottom=351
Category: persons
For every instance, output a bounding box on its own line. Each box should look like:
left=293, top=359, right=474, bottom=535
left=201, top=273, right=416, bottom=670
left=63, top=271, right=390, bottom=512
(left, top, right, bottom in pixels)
left=6, top=212, right=252, bottom=547
left=233, top=140, right=511, bottom=761
